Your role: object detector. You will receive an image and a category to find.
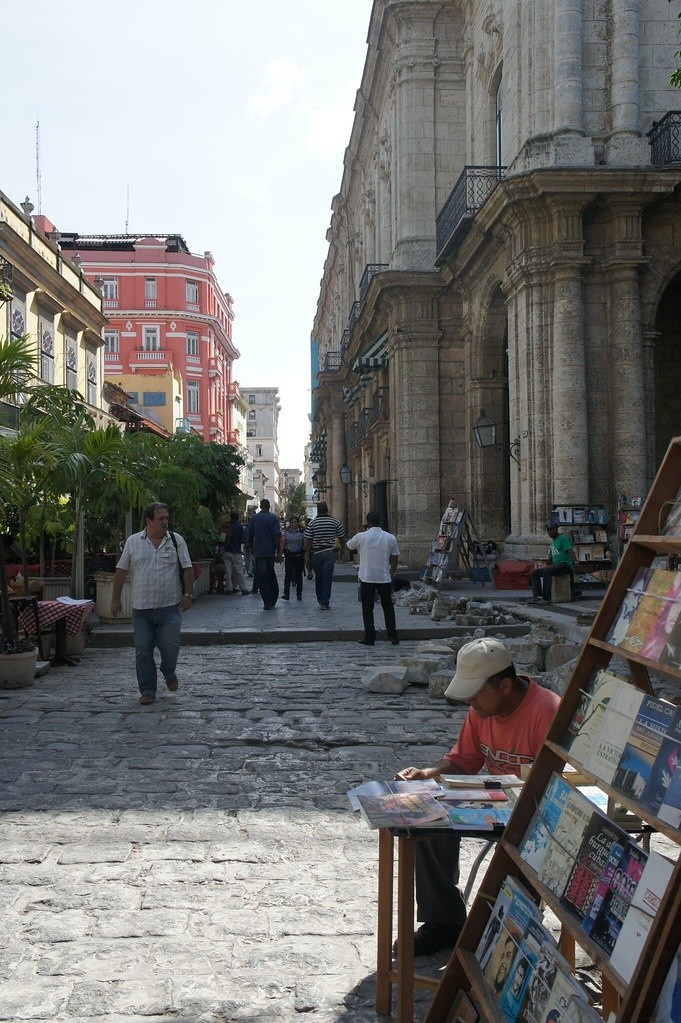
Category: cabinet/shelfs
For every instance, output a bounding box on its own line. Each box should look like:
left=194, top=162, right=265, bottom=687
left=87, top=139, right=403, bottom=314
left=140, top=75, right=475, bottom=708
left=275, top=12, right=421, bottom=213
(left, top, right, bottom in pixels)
left=618, top=497, right=646, bottom=560
left=551, top=505, right=612, bottom=566
left=427, top=442, right=681, bottom=1023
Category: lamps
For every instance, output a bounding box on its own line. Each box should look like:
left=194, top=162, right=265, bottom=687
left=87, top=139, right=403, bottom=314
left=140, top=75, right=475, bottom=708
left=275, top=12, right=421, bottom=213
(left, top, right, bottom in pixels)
left=339, top=464, right=369, bottom=497
left=312, top=466, right=332, bottom=492
left=473, top=409, right=521, bottom=471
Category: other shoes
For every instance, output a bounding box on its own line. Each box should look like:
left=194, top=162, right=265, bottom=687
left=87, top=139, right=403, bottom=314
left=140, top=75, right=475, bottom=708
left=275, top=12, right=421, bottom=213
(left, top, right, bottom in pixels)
left=242, top=589, right=250, bottom=594
left=393, top=920, right=464, bottom=957
left=321, top=604, right=331, bottom=610
left=166, top=674, right=179, bottom=691
left=297, top=594, right=302, bottom=600
left=281, top=594, right=289, bottom=600
left=358, top=638, right=375, bottom=645
left=141, top=694, right=156, bottom=704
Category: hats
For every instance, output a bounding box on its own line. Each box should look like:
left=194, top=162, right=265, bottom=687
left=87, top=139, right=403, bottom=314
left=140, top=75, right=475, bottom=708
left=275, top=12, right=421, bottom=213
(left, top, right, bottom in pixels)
left=443, top=637, right=512, bottom=699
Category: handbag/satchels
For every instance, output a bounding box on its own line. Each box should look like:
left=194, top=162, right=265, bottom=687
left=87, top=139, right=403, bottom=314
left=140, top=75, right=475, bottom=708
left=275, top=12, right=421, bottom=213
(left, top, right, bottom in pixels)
left=286, top=554, right=303, bottom=566
left=218, top=521, right=232, bottom=545
left=180, top=562, right=201, bottom=593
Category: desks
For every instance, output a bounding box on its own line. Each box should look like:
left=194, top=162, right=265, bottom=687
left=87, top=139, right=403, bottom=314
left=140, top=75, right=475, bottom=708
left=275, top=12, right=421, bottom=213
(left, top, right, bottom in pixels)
left=18, top=596, right=95, bottom=666
left=363, top=766, right=528, bottom=1022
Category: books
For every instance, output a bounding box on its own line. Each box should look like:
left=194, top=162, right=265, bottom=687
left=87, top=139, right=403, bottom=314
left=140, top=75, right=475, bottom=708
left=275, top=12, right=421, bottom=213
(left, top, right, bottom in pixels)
left=659, top=482, right=681, bottom=536
left=419, top=508, right=463, bottom=582
left=346, top=762, right=533, bottom=831
left=552, top=508, right=615, bottom=582
left=446, top=551, right=681, bottom=1023
left=620, top=496, right=646, bottom=556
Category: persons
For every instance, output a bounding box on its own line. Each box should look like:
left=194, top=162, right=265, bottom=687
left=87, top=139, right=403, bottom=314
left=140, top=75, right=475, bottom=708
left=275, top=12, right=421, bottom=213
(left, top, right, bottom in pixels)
left=393, top=638, right=561, bottom=959
left=441, top=800, right=493, bottom=809
left=517, top=960, right=548, bottom=1017
left=520, top=822, right=551, bottom=873
left=479, top=905, right=503, bottom=964
left=248, top=499, right=281, bottom=610
left=279, top=516, right=304, bottom=601
left=304, top=502, right=346, bottom=610
left=346, top=511, right=401, bottom=646
left=213, top=513, right=251, bottom=595
left=604, top=568, right=649, bottom=646
left=298, top=518, right=314, bottom=580
left=110, top=502, right=195, bottom=704
left=243, top=516, right=260, bottom=594
left=641, top=584, right=681, bottom=662
left=509, top=958, right=529, bottom=997
left=526, top=522, right=577, bottom=605
left=494, top=933, right=519, bottom=993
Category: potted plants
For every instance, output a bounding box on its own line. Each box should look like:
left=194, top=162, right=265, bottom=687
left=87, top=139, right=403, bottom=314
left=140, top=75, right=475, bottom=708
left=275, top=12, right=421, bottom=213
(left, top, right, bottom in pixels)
left=0, top=638, right=40, bottom=689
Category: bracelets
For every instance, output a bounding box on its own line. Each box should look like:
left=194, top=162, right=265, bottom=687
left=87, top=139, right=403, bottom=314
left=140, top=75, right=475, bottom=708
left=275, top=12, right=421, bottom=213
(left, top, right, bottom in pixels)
left=184, top=592, right=193, bottom=601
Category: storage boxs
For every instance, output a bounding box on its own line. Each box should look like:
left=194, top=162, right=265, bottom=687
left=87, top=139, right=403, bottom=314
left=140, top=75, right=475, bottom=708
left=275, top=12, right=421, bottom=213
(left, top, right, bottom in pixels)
left=497, top=560, right=531, bottom=589
left=471, top=568, right=492, bottom=582
